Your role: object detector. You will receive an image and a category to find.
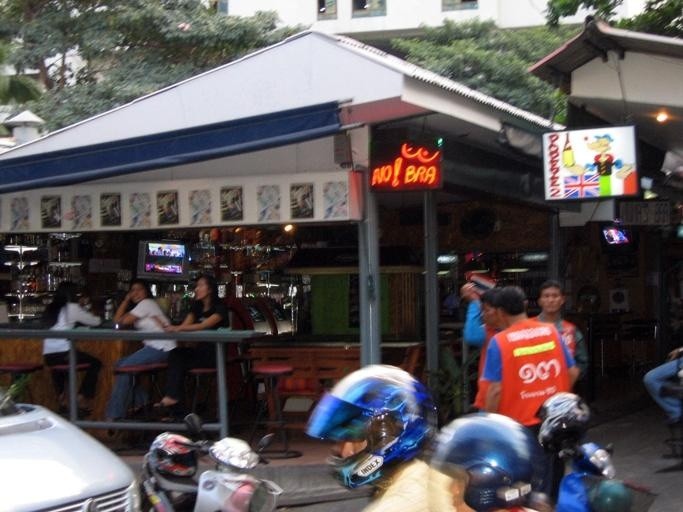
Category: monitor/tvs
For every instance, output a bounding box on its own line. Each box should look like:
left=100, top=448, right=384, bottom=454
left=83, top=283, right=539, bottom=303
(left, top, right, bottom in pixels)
left=137, top=240, right=192, bottom=282
left=598, top=222, right=640, bottom=254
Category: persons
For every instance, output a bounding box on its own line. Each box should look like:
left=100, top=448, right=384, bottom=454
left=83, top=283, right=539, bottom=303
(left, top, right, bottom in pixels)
left=40, top=281, right=101, bottom=414
left=642, top=346, right=683, bottom=430
left=146, top=242, right=183, bottom=273
left=304, top=363, right=593, bottom=512
left=105, top=276, right=230, bottom=424
left=458, top=272, right=589, bottom=438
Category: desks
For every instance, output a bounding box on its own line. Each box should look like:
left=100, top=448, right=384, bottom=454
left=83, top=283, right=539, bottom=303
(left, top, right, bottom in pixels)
left=0, top=328, right=265, bottom=438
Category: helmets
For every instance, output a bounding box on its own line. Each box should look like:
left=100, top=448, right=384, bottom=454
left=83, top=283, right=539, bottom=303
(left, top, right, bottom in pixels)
left=303, top=364, right=437, bottom=487
left=533, top=390, right=592, bottom=449
left=150, top=429, right=200, bottom=479
left=433, top=412, right=549, bottom=512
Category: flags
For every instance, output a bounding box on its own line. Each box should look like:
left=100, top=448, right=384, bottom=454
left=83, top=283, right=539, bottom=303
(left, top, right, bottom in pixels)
left=564, top=173, right=600, bottom=199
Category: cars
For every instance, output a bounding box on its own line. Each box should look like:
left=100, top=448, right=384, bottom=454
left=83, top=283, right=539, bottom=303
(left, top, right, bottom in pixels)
left=0, top=403, right=142, bottom=512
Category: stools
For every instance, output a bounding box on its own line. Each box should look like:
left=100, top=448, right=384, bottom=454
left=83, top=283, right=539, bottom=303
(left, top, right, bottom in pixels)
left=251, top=364, right=297, bottom=454
left=111, top=362, right=175, bottom=444
left=190, top=366, right=234, bottom=439
left=54, top=359, right=97, bottom=426
left=1, top=359, right=48, bottom=420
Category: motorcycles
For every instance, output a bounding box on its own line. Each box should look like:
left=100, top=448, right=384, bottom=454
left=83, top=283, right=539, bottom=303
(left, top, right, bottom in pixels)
left=143, top=413, right=283, bottom=512
left=538, top=420, right=618, bottom=512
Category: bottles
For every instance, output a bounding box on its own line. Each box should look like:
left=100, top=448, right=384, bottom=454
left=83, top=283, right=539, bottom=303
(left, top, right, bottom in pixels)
left=561, top=131, right=576, bottom=170
left=9, top=266, right=72, bottom=296
